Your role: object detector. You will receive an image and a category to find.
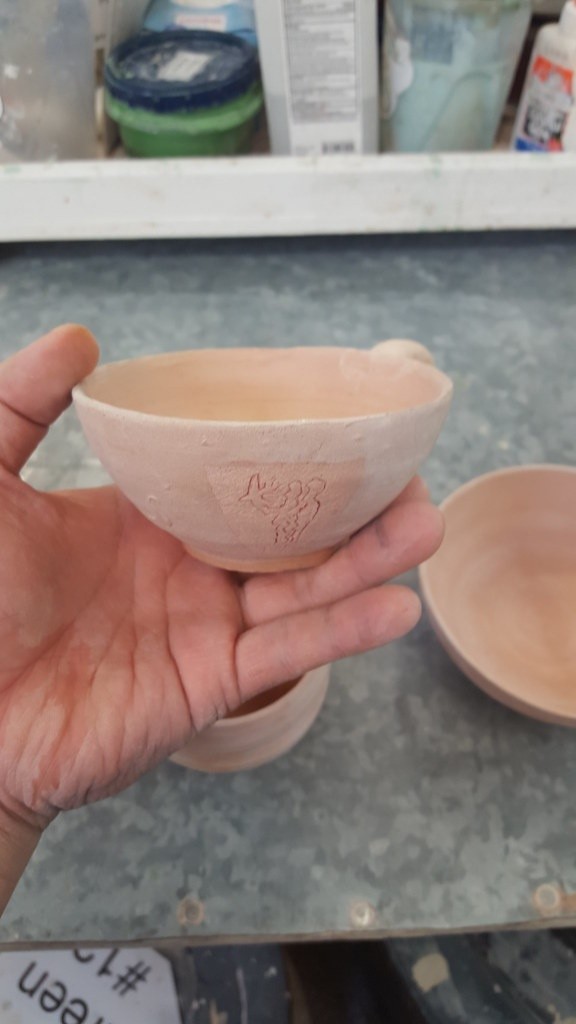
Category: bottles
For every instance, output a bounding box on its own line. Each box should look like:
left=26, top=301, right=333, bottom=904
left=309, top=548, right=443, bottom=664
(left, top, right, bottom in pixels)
left=507, top=0, right=576, bottom=152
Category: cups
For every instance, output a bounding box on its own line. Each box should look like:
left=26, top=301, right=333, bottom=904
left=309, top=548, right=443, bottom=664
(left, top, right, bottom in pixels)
left=377, top=0, right=532, bottom=151
left=162, top=663, right=329, bottom=773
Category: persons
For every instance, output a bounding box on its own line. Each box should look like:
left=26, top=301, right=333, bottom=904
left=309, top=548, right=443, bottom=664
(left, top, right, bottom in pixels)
left=0, top=322, right=450, bottom=918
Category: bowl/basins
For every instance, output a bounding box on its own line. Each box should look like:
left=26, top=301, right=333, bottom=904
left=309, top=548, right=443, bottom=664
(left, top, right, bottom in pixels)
left=415, top=465, right=576, bottom=725
left=70, top=347, right=452, bottom=575
